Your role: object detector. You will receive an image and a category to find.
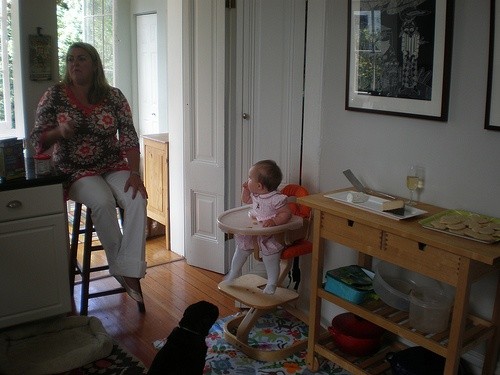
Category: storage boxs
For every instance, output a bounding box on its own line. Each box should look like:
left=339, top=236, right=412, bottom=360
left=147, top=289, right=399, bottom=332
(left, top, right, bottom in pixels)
left=0, top=139, right=26, bottom=180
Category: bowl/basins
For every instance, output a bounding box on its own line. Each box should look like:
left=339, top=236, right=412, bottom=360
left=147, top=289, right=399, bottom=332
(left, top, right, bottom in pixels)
left=385, top=346, right=469, bottom=375
left=373, top=261, right=442, bottom=313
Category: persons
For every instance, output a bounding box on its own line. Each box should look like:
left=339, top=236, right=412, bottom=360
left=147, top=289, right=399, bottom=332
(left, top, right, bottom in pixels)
left=29, top=42, right=148, bottom=303
left=224, top=159, right=291, bottom=296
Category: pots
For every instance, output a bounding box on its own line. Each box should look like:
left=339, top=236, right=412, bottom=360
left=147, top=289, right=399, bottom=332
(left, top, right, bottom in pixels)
left=328, top=313, right=386, bottom=357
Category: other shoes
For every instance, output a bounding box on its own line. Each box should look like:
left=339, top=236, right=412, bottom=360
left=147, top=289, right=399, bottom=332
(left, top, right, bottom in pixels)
left=114, top=275, right=142, bottom=304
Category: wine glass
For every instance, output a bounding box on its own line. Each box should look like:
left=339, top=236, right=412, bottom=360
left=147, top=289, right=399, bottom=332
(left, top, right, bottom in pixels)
left=405, top=163, right=418, bottom=206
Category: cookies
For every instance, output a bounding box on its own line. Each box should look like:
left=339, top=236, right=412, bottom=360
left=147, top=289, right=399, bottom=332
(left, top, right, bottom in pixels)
left=431, top=214, right=500, bottom=242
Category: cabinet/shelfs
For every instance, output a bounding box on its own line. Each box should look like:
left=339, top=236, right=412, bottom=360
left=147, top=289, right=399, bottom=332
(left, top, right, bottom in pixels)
left=296, top=186, right=500, bottom=375
left=143, top=134, right=173, bottom=254
left=0, top=175, right=73, bottom=331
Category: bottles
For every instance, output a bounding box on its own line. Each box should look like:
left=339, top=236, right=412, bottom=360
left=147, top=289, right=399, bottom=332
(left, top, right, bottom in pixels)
left=33, top=155, right=50, bottom=175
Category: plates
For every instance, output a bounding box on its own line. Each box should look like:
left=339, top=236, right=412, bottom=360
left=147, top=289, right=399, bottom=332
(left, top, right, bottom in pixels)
left=417, top=209, right=500, bottom=244
left=323, top=191, right=428, bottom=221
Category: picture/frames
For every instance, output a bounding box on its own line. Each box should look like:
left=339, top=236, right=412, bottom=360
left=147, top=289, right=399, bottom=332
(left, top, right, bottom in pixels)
left=484, top=0, right=500, bottom=132
left=344, top=0, right=456, bottom=122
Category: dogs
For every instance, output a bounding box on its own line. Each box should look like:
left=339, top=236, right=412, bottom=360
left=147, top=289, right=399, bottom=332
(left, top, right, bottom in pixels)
left=144, top=299, right=221, bottom=375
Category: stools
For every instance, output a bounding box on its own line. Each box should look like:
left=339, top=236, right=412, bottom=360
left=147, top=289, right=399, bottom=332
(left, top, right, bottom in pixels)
left=69, top=200, right=147, bottom=317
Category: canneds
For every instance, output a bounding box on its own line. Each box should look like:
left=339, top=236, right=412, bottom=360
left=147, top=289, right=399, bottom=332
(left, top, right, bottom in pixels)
left=33, top=154, right=51, bottom=175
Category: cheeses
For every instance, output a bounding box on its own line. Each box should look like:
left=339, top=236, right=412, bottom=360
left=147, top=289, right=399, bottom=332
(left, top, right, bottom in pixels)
left=381, top=199, right=405, bottom=215
left=347, top=192, right=367, bottom=202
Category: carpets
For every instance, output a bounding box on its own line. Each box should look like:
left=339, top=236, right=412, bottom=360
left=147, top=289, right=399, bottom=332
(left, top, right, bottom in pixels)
left=152, top=306, right=348, bottom=375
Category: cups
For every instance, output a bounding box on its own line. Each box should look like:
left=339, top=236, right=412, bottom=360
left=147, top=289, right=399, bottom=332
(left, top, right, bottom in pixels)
left=408, top=287, right=453, bottom=333
